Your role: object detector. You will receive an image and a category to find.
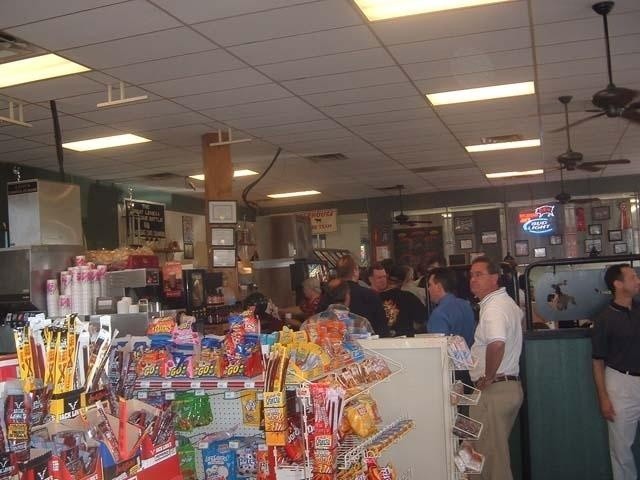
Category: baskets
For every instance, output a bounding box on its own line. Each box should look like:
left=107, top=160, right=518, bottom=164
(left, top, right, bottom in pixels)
left=451, top=413, right=483, bottom=438
left=449, top=380, right=482, bottom=405
left=463, top=450, right=486, bottom=474
left=447, top=353, right=481, bottom=372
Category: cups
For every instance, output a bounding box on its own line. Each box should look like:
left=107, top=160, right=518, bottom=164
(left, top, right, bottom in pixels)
left=117, top=297, right=139, bottom=313
left=47, top=255, right=108, bottom=317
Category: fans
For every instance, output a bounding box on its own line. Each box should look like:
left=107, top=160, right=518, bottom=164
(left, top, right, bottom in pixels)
left=379, top=184, right=432, bottom=225
left=538, top=1, right=640, bottom=209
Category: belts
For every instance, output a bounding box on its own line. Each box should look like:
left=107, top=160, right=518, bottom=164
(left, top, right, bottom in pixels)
left=473, top=373, right=519, bottom=387
left=606, top=364, right=639, bottom=376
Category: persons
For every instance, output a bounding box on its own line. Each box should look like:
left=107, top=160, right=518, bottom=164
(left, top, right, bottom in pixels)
left=243, top=282, right=268, bottom=316
left=468, top=257, right=524, bottom=480
left=590, top=263, right=640, bottom=480
left=299, top=256, right=526, bottom=338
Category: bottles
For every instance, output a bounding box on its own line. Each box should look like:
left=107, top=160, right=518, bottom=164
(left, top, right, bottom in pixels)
left=2, top=321, right=67, bottom=350
left=192, top=307, right=230, bottom=324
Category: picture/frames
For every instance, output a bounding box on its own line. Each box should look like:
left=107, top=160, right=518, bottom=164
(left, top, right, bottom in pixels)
left=514, top=205, right=629, bottom=258
left=207, top=200, right=238, bottom=268
left=449, top=215, right=498, bottom=266
